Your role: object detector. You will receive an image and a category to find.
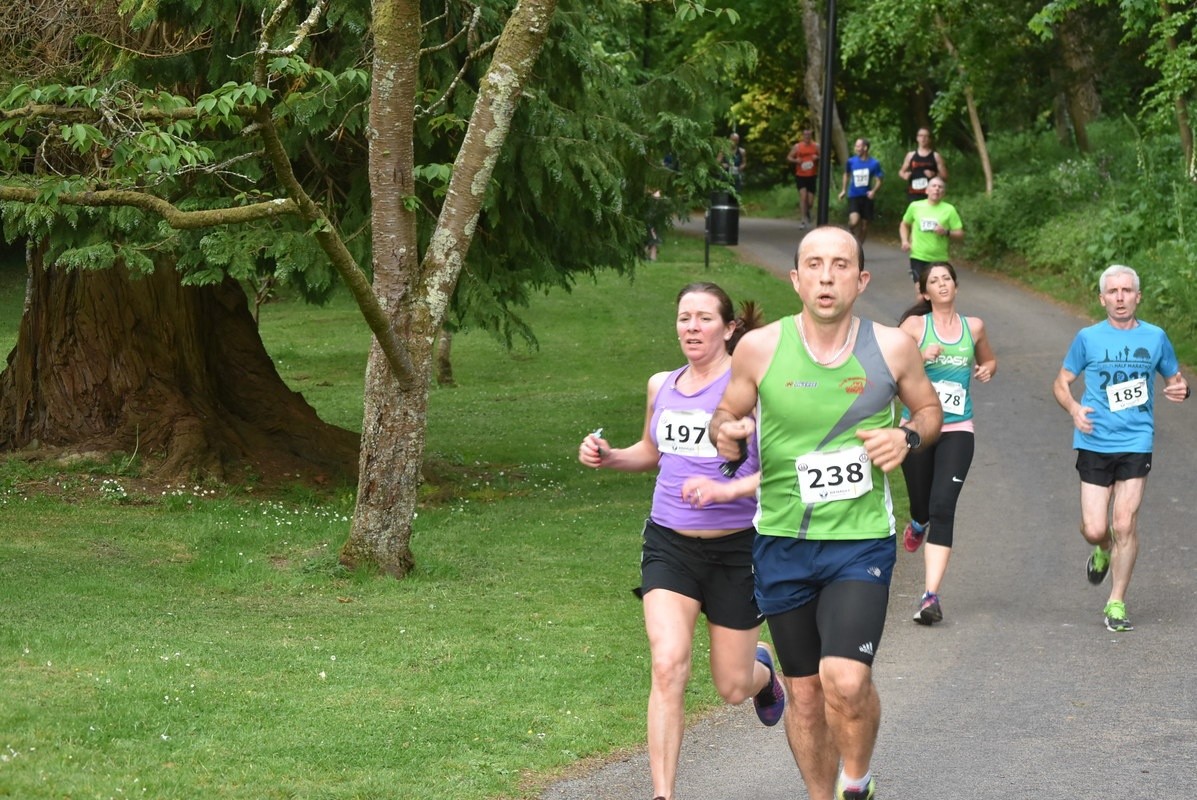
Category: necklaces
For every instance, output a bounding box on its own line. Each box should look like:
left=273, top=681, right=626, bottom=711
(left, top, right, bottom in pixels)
left=799, top=313, right=855, bottom=366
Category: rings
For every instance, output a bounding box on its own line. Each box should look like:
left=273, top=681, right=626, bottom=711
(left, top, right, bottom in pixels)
left=697, top=489, right=702, bottom=499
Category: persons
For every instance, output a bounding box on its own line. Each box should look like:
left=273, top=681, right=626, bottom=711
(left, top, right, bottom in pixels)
left=787, top=127, right=821, bottom=230
left=899, top=126, right=950, bottom=274
left=839, top=138, right=884, bottom=244
left=899, top=177, right=965, bottom=304
left=1053, top=265, right=1190, bottom=632
left=644, top=151, right=682, bottom=261
left=708, top=224, right=943, bottom=800
left=716, top=133, right=746, bottom=196
left=898, top=261, right=997, bottom=626
left=579, top=282, right=786, bottom=800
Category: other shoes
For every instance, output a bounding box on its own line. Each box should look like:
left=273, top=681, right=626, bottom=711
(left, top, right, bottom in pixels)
left=835, top=771, right=875, bottom=800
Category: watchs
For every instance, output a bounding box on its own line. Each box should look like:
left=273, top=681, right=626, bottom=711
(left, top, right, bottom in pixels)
left=897, top=426, right=921, bottom=454
left=1185, top=386, right=1190, bottom=399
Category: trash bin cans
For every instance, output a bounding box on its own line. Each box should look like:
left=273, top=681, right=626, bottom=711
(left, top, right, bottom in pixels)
left=708, top=191, right=740, bottom=247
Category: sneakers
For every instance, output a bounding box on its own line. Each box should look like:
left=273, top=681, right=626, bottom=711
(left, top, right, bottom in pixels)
left=1104, top=599, right=1133, bottom=632
left=753, top=641, right=786, bottom=727
left=913, top=592, right=942, bottom=627
left=904, top=521, right=926, bottom=552
left=1085, top=527, right=1115, bottom=585
